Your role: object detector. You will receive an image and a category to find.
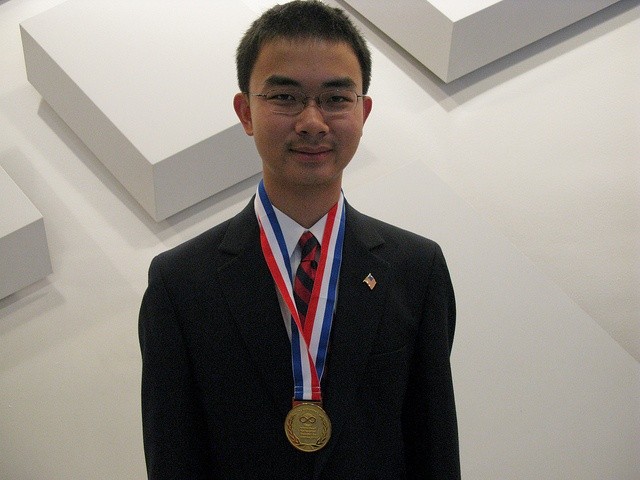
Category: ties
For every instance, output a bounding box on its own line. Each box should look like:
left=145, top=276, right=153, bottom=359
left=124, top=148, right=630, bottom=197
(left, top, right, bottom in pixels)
left=294, top=232, right=321, bottom=329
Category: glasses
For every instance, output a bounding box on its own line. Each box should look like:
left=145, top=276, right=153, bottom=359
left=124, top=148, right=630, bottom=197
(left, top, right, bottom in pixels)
left=241, top=88, right=368, bottom=115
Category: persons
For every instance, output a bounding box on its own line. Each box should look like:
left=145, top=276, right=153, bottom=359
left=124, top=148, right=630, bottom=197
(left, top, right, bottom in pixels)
left=138, top=0, right=462, bottom=480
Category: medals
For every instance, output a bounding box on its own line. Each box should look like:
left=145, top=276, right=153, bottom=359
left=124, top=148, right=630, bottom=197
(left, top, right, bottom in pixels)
left=253, top=180, right=347, bottom=453
